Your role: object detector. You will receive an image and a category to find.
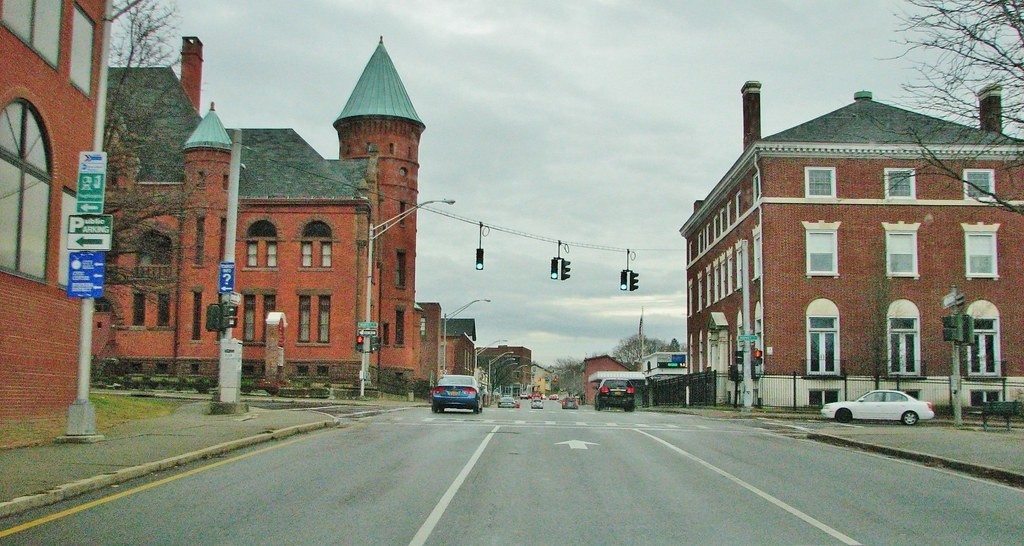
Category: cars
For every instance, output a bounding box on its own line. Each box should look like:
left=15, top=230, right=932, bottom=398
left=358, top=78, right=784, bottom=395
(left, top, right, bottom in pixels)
left=562, top=398, right=579, bottom=409
left=530, top=399, right=544, bottom=409
left=820, top=389, right=934, bottom=428
left=520, top=392, right=559, bottom=401
left=432, top=374, right=483, bottom=414
left=498, top=395, right=515, bottom=408
left=593, top=378, right=635, bottom=412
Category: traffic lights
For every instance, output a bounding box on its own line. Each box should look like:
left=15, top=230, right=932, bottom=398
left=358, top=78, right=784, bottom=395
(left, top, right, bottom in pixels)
left=941, top=315, right=963, bottom=342
left=551, top=259, right=558, bottom=280
left=221, top=305, right=237, bottom=331
left=621, top=272, right=627, bottom=290
left=561, top=258, right=570, bottom=280
left=356, top=336, right=363, bottom=352
left=476, top=249, right=484, bottom=270
left=630, top=272, right=639, bottom=291
left=554, top=376, right=557, bottom=382
left=755, top=350, right=762, bottom=365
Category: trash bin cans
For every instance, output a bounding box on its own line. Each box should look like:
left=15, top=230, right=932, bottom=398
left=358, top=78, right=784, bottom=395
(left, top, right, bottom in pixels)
left=408, top=390, right=414, bottom=402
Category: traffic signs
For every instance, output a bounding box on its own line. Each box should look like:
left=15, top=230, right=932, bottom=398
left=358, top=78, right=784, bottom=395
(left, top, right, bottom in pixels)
left=67, top=214, right=112, bottom=251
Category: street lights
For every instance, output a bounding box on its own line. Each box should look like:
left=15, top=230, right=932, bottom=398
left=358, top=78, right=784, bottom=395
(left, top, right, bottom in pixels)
left=443, top=299, right=528, bottom=393
left=359, top=198, right=457, bottom=396
